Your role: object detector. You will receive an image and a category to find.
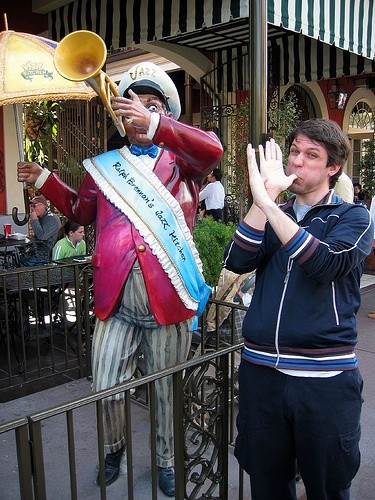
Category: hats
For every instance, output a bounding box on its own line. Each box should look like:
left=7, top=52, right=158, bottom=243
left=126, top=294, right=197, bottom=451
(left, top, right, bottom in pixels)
left=29, top=196, right=48, bottom=206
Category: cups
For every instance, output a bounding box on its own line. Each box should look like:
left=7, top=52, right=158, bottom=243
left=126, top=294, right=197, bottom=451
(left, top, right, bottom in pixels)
left=3, top=224, right=12, bottom=236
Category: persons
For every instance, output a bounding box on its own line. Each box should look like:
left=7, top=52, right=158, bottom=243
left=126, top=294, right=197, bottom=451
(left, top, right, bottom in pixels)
left=197, top=168, right=226, bottom=222
left=19, top=196, right=62, bottom=267
left=333, top=171, right=355, bottom=202
left=353, top=182, right=366, bottom=201
left=223, top=117, right=375, bottom=500
left=17, top=61, right=224, bottom=496
left=49, top=221, right=87, bottom=261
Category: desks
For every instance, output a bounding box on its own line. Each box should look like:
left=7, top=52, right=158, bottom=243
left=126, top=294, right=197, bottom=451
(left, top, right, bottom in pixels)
left=0, top=234, right=94, bottom=373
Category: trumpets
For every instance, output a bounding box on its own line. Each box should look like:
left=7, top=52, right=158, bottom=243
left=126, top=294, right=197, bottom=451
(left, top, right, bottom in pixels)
left=53, top=30, right=126, bottom=138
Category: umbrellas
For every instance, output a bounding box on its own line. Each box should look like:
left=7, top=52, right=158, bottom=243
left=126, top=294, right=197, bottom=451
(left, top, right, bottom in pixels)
left=0, top=13, right=101, bottom=227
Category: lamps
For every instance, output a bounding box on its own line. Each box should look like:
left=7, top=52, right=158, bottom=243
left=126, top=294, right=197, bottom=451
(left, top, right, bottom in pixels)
left=327, top=78, right=350, bottom=109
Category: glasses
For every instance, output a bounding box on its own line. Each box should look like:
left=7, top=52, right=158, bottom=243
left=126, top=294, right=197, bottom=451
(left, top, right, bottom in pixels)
left=30, top=203, right=43, bottom=208
